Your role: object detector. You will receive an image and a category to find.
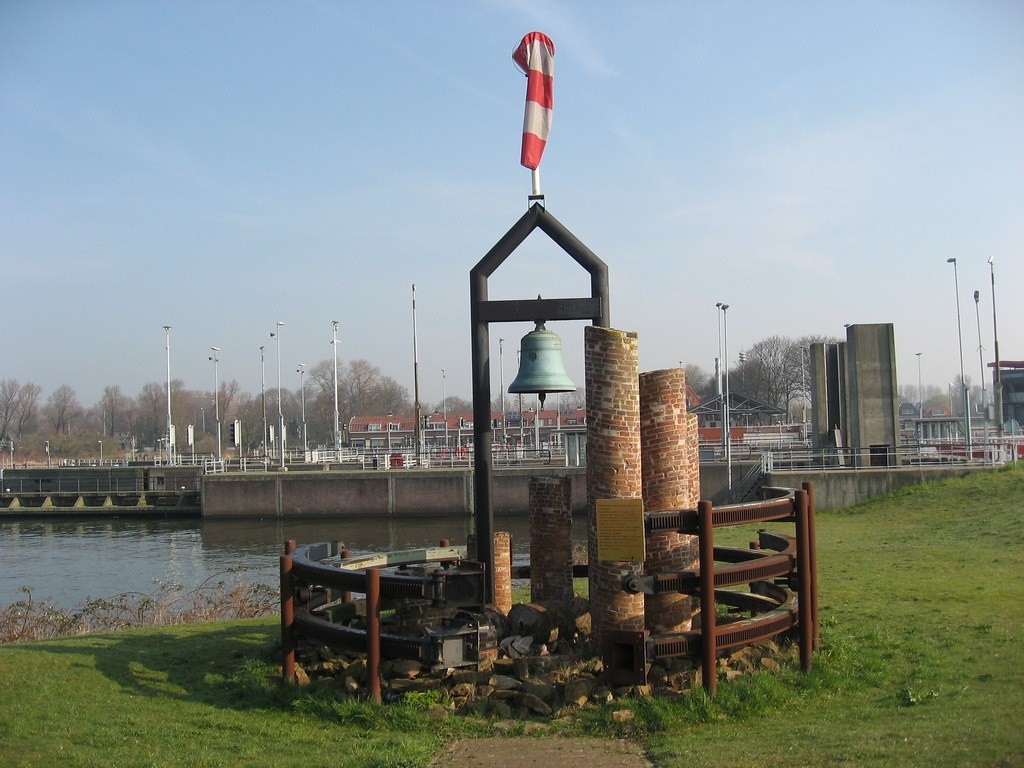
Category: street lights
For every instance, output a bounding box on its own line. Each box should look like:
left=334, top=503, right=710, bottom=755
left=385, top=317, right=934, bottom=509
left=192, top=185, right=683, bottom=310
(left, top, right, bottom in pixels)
left=163, top=325, right=171, bottom=436
left=276, top=322, right=284, bottom=456
left=947, top=258, right=968, bottom=387
left=45, top=441, right=49, bottom=456
left=330, top=320, right=342, bottom=451
left=297, top=364, right=305, bottom=422
left=715, top=302, right=724, bottom=396
left=98, top=441, right=102, bottom=460
left=210, top=346, right=220, bottom=421
left=917, top=353, right=925, bottom=439
left=721, top=304, right=733, bottom=491
left=441, top=369, right=446, bottom=421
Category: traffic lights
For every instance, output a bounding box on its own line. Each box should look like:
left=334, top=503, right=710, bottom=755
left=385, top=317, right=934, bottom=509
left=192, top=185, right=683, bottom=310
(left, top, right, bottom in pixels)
left=461, top=419, right=465, bottom=427
left=230, top=423, right=234, bottom=443
